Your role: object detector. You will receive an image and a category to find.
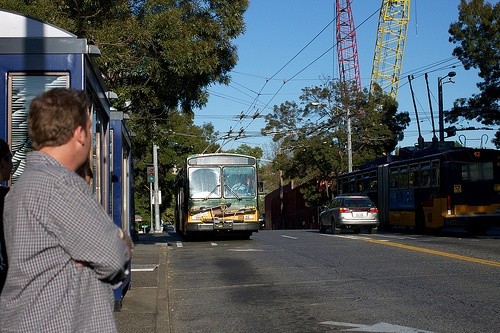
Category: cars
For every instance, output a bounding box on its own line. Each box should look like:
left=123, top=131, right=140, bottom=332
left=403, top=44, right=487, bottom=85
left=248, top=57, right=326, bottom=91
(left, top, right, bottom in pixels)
left=259, top=213, right=266, bottom=230
left=318, top=194, right=379, bottom=234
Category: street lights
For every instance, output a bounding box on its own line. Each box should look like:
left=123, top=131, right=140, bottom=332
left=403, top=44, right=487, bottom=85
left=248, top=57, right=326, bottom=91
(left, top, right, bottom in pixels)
left=436, top=71, right=456, bottom=140
left=312, top=102, right=352, bottom=172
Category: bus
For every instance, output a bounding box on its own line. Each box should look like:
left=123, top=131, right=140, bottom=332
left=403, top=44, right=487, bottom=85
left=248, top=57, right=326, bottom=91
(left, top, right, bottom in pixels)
left=174, top=153, right=260, bottom=242
left=332, top=143, right=496, bottom=237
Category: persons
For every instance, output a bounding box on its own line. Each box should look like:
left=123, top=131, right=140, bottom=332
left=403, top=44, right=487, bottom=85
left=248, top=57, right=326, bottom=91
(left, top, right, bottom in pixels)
left=0, top=87, right=129, bottom=333
left=75, top=157, right=94, bottom=185
left=1, top=137, right=14, bottom=292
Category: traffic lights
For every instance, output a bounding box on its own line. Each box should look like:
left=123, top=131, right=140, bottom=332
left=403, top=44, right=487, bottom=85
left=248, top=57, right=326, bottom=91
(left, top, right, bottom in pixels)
left=147, top=166, right=157, bottom=235
